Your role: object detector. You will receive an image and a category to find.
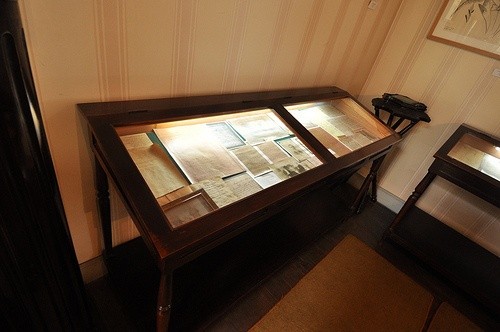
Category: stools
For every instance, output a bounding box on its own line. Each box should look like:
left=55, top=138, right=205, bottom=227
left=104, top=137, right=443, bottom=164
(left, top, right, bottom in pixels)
left=354, top=93, right=431, bottom=213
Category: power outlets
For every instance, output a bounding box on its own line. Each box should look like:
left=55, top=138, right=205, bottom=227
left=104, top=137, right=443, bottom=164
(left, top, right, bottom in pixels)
left=367, top=1, right=377, bottom=11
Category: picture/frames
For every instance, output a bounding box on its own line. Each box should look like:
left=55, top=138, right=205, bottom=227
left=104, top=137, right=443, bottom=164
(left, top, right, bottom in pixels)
left=427, top=0, right=500, bottom=62
